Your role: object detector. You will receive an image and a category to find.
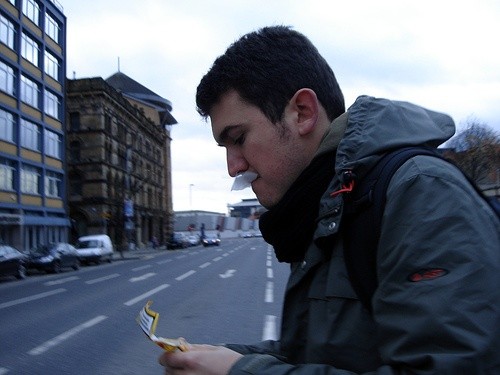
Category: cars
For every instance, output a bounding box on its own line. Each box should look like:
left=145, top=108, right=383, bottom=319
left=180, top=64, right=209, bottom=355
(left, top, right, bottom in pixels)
left=185, top=232, right=200, bottom=246
left=241, top=229, right=254, bottom=238
left=0, top=244, right=31, bottom=279
left=166, top=232, right=190, bottom=249
left=203, top=233, right=221, bottom=247
left=254, top=230, right=263, bottom=237
left=26, top=242, right=80, bottom=273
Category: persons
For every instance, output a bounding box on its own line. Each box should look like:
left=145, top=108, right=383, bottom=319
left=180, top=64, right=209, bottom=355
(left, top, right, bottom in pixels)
left=158, top=26, right=500, bottom=375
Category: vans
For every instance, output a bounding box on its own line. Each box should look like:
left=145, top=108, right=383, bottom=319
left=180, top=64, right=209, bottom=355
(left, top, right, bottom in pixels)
left=77, top=234, right=114, bottom=265
